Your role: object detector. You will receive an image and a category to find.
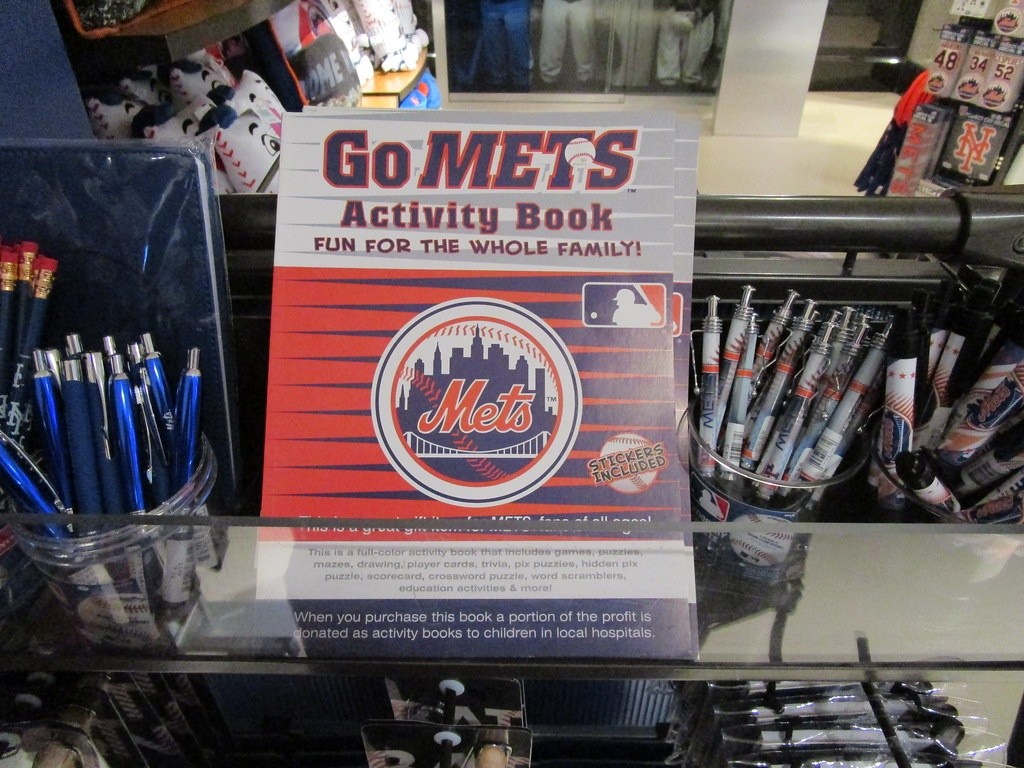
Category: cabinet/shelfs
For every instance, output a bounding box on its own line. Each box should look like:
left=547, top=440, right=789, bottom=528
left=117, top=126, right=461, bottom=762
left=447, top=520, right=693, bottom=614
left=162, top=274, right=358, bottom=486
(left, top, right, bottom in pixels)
left=47, top=0, right=427, bottom=186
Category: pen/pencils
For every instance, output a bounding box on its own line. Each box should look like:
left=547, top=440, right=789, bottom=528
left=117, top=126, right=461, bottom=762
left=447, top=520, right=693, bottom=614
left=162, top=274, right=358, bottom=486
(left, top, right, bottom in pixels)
left=0, top=239, right=58, bottom=516
left=691, top=286, right=894, bottom=512
left=0, top=332, right=222, bottom=621
left=878, top=265, right=1024, bottom=524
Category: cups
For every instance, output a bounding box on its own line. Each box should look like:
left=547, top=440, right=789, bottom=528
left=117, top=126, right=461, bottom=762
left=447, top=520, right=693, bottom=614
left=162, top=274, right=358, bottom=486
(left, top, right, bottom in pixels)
left=10, top=433, right=218, bottom=649
left=866, top=396, right=1024, bottom=528
left=689, top=399, right=873, bottom=522
